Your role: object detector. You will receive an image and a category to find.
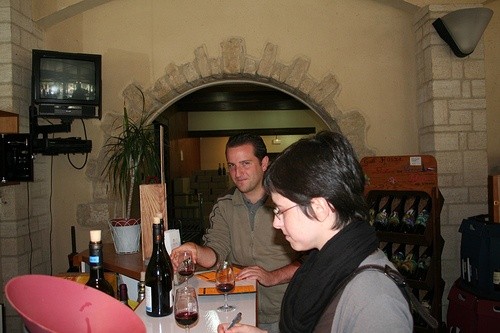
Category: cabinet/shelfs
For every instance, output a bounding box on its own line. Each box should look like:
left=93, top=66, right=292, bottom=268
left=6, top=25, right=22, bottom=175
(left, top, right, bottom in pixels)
left=359, top=155, right=447, bottom=333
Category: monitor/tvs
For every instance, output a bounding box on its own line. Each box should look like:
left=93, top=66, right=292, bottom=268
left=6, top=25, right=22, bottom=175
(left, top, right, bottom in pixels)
left=32, top=48, right=101, bottom=105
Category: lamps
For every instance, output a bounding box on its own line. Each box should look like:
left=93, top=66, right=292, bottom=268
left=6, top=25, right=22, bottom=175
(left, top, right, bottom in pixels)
left=272, top=136, right=281, bottom=145
left=432, top=8, right=494, bottom=58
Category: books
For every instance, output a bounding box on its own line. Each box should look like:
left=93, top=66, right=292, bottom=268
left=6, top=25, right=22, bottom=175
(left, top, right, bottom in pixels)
left=192, top=264, right=257, bottom=296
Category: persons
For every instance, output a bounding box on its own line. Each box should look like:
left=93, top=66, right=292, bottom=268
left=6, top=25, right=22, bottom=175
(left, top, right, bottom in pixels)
left=217, top=129, right=415, bottom=333
left=169, top=134, right=319, bottom=332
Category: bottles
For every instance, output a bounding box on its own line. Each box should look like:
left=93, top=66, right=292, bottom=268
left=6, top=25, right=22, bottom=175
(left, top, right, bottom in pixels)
left=367, top=196, right=382, bottom=228
left=222, top=163, right=227, bottom=175
left=380, top=240, right=392, bottom=261
left=137, top=272, right=147, bottom=302
left=218, top=163, right=222, bottom=175
left=84, top=229, right=115, bottom=298
left=389, top=240, right=406, bottom=269
left=156, top=214, right=174, bottom=279
left=419, top=287, right=435, bottom=314
left=415, top=242, right=433, bottom=280
left=400, top=196, right=421, bottom=234
left=388, top=195, right=406, bottom=232
left=399, top=242, right=420, bottom=275
left=413, top=197, right=432, bottom=234
left=118, top=284, right=133, bottom=311
left=144, top=217, right=174, bottom=317
left=411, top=286, right=420, bottom=300
left=374, top=195, right=396, bottom=229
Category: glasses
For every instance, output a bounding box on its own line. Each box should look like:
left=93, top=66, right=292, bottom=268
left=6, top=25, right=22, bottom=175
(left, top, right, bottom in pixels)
left=272, top=199, right=303, bottom=219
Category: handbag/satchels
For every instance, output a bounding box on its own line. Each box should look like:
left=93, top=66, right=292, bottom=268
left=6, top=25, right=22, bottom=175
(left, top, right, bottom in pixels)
left=346, top=265, right=451, bottom=332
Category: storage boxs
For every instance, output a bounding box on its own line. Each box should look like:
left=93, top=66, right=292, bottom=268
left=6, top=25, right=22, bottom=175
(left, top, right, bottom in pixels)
left=175, top=169, right=232, bottom=228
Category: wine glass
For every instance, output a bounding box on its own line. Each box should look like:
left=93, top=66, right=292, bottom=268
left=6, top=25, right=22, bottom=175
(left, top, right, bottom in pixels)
left=176, top=250, right=196, bottom=288
left=215, top=261, right=237, bottom=313
left=174, top=288, right=200, bottom=333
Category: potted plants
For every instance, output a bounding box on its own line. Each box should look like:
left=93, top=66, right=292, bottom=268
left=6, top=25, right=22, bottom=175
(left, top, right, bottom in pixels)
left=101, top=84, right=170, bottom=253
